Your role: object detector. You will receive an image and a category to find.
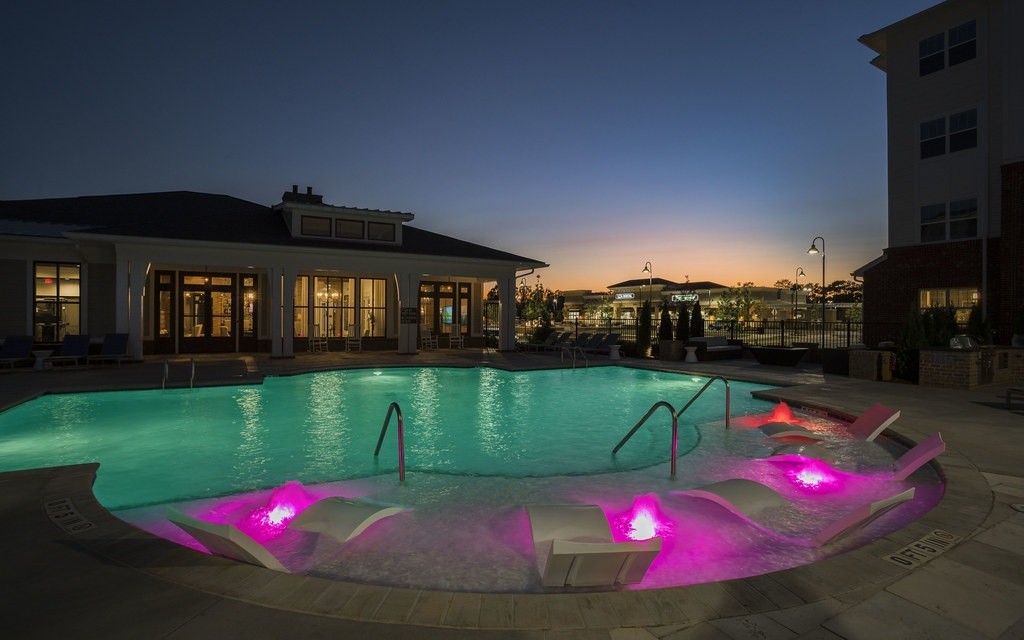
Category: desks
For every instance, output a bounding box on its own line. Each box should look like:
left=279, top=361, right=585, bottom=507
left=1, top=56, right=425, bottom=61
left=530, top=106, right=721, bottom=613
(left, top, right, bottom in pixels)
left=751, top=346, right=809, bottom=366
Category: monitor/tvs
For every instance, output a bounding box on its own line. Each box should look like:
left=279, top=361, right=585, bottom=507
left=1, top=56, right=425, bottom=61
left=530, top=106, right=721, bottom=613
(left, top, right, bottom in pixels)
left=441, top=306, right=453, bottom=324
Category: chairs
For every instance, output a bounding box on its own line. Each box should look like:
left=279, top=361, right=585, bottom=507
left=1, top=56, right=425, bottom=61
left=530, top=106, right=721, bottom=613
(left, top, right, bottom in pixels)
left=309, top=326, right=327, bottom=352
left=0, top=334, right=128, bottom=374
left=674, top=479, right=918, bottom=548
left=527, top=501, right=660, bottom=591
left=347, top=325, right=362, bottom=352
left=449, top=324, right=464, bottom=349
left=768, top=431, right=946, bottom=481
left=420, top=324, right=440, bottom=351
left=759, top=402, right=900, bottom=441
left=168, top=495, right=417, bottom=572
left=525, top=332, right=621, bottom=358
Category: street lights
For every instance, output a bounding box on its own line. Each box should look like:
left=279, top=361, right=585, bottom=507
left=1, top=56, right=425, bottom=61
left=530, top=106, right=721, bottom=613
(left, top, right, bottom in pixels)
left=519, top=278, right=527, bottom=336
left=794, top=267, right=806, bottom=335
left=806, top=236, right=825, bottom=348
left=641, top=261, right=653, bottom=341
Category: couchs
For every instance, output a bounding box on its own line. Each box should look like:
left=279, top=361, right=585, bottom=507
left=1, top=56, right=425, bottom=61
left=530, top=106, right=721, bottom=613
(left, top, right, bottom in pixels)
left=688, top=336, right=744, bottom=361
left=822, top=344, right=869, bottom=375
